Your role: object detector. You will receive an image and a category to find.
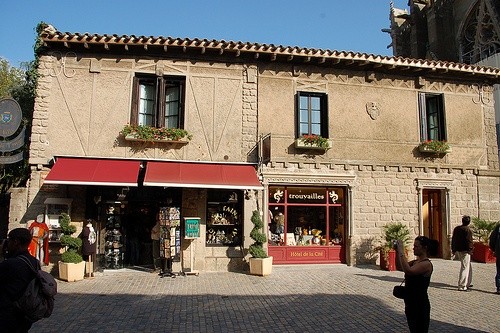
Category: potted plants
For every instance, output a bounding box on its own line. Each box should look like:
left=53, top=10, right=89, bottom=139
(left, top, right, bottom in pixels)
left=249, top=211, right=272, bottom=275
left=58, top=213, right=86, bottom=282
left=380, top=221, right=411, bottom=270
left=473, top=217, right=497, bottom=263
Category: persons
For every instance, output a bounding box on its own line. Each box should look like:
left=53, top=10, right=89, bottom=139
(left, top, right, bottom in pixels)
left=392, top=236, right=440, bottom=333
left=274, top=212, right=285, bottom=233
left=0, top=228, right=42, bottom=333
left=28, top=213, right=49, bottom=267
left=78, top=223, right=96, bottom=256
left=488, top=220, right=500, bottom=294
left=450, top=215, right=473, bottom=293
left=268, top=209, right=274, bottom=223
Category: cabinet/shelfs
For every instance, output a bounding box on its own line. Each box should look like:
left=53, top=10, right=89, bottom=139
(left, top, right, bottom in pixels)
left=159, top=206, right=182, bottom=278
left=43, top=198, right=71, bottom=252
left=103, top=200, right=126, bottom=269
left=207, top=202, right=241, bottom=246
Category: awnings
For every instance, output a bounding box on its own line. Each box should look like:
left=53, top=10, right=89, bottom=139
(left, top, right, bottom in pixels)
left=143, top=162, right=264, bottom=190
left=43, top=158, right=141, bottom=186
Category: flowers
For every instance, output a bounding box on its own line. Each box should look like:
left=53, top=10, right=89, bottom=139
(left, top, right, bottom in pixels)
left=118, top=122, right=192, bottom=154
left=303, top=135, right=330, bottom=155
left=422, top=141, right=449, bottom=157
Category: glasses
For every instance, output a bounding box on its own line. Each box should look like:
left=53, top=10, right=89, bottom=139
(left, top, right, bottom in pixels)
left=419, top=236, right=423, bottom=241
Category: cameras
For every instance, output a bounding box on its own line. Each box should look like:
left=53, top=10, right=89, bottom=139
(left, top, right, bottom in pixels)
left=393, top=239, right=398, bottom=247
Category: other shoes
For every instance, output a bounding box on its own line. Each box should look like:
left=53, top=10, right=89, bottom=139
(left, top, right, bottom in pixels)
left=497, top=290, right=500, bottom=295
left=458, top=285, right=473, bottom=291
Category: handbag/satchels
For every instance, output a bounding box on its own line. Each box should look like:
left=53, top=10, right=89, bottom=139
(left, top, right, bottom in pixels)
left=393, top=284, right=407, bottom=300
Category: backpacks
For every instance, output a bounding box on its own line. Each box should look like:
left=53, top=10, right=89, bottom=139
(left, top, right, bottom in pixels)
left=11, top=254, right=58, bottom=324
